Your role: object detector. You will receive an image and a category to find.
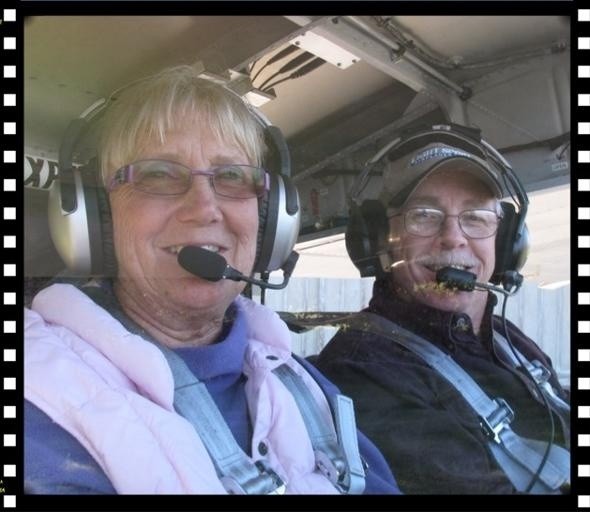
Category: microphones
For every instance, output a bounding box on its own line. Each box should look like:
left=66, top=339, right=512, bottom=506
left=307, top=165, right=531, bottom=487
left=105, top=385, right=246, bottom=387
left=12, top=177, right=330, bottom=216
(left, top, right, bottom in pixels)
left=436, top=267, right=523, bottom=296
left=178, top=246, right=299, bottom=289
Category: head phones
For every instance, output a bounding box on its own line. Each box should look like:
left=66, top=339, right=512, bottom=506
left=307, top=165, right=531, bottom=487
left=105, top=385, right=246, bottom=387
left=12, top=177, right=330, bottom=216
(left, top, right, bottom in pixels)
left=345, top=123, right=530, bottom=278
left=48, top=65, right=302, bottom=279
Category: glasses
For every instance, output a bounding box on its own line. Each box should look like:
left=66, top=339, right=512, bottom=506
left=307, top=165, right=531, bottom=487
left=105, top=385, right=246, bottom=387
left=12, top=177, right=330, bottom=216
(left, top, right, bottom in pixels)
left=105, top=158, right=271, bottom=199
left=387, top=208, right=504, bottom=238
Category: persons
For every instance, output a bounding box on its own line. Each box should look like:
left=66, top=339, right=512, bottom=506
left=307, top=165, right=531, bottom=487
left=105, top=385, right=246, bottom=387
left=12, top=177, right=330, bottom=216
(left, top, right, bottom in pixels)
left=24, top=65, right=402, bottom=495
left=313, top=138, right=571, bottom=495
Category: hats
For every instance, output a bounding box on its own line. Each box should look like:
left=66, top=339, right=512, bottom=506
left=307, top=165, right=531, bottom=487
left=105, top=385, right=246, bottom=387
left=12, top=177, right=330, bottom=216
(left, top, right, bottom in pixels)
left=380, top=142, right=503, bottom=207
